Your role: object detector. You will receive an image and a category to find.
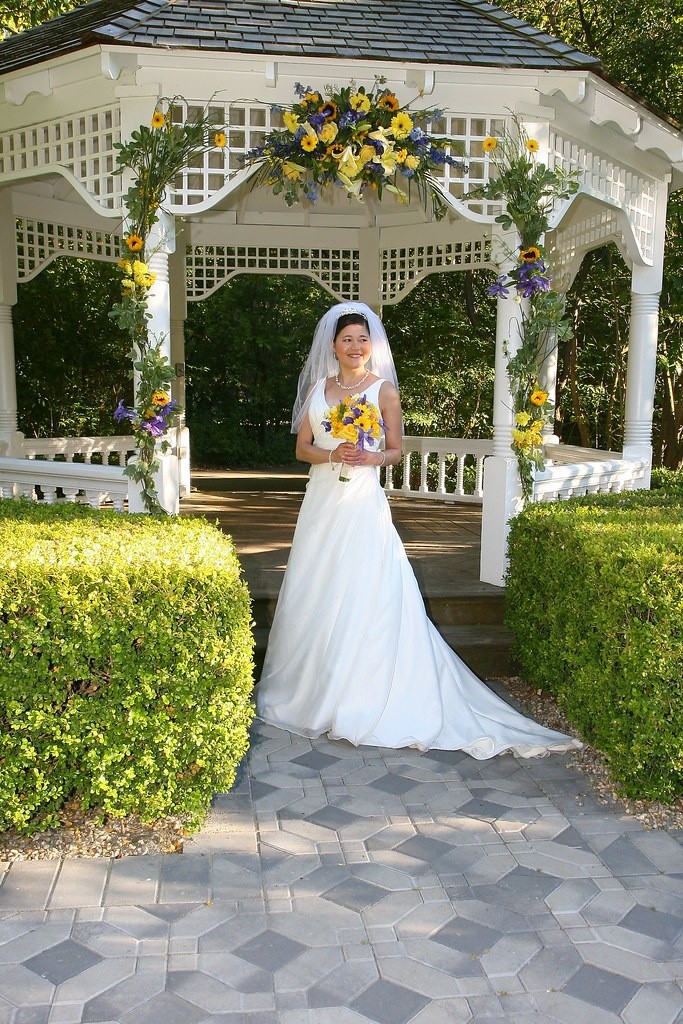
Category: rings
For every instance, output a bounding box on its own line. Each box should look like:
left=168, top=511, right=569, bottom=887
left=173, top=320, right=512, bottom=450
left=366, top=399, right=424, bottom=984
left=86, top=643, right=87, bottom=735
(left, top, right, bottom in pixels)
left=357, top=461, right=360, bottom=465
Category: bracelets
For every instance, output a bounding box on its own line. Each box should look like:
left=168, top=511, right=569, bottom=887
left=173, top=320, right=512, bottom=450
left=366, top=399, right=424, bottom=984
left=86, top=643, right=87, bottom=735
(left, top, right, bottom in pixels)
left=378, top=452, right=386, bottom=467
left=328, top=449, right=340, bottom=471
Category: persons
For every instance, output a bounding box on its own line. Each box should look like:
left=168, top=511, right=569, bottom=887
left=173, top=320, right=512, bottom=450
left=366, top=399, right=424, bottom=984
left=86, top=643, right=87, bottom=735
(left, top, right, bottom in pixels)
left=255, top=302, right=404, bottom=748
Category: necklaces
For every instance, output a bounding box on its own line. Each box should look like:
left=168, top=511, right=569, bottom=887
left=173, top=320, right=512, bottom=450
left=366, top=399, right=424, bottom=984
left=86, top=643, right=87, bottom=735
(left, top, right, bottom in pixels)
left=335, top=367, right=370, bottom=389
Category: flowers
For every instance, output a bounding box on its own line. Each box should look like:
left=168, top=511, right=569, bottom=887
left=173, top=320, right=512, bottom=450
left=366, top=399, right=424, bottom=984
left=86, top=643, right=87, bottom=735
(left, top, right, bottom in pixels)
left=474, top=125, right=577, bottom=501
left=234, top=76, right=472, bottom=219
left=107, top=98, right=228, bottom=516
left=322, top=394, right=389, bottom=483
